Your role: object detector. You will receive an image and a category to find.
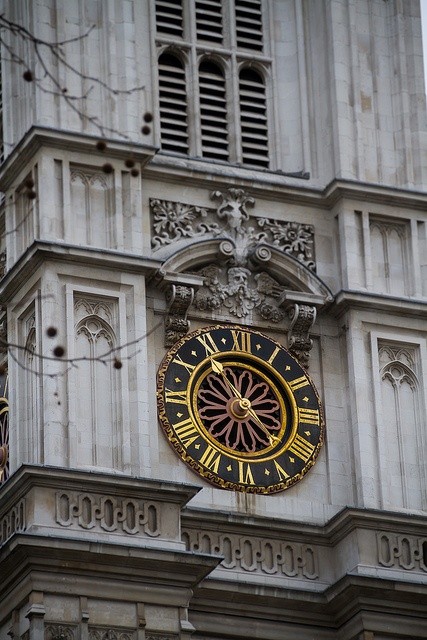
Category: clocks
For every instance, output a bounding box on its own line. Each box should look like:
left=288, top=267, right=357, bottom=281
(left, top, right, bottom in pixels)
left=157, top=325, right=328, bottom=495
